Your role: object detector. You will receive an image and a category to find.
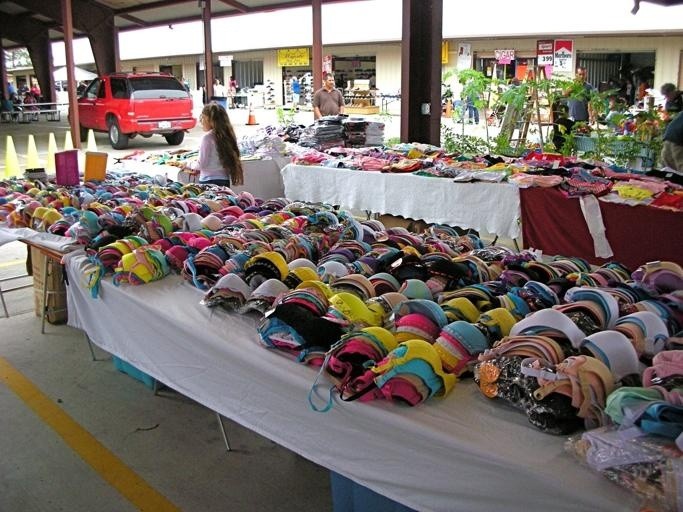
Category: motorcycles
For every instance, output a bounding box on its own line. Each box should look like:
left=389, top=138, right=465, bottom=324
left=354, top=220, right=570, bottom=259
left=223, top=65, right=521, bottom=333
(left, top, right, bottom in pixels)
left=11, top=91, right=48, bottom=124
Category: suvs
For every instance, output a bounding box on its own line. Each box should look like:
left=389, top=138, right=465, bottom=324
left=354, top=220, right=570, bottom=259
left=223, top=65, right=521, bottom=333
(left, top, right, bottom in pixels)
left=66, top=66, right=196, bottom=150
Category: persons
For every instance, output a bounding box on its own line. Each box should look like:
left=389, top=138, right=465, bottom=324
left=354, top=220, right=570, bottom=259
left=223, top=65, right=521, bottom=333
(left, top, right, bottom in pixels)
left=185, top=103, right=245, bottom=189
left=292, top=76, right=302, bottom=110
left=449, top=65, right=682, bottom=178
left=0, top=75, right=242, bottom=123
left=312, top=72, right=345, bottom=121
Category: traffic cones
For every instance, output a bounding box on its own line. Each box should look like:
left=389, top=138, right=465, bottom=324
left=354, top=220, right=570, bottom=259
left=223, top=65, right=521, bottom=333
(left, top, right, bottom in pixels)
left=63, top=129, right=81, bottom=174
left=245, top=101, right=259, bottom=126
left=84, top=128, right=100, bottom=155
left=45, top=132, right=61, bottom=175
left=2, top=135, right=21, bottom=182
left=23, top=134, right=43, bottom=175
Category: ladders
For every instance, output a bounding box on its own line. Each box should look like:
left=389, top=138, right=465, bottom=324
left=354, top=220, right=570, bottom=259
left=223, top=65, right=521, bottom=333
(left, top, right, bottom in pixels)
left=508, top=65, right=553, bottom=153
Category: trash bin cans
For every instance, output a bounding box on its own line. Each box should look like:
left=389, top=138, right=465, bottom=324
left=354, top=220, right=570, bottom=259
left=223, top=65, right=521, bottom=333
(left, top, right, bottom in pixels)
left=211, top=96, right=227, bottom=111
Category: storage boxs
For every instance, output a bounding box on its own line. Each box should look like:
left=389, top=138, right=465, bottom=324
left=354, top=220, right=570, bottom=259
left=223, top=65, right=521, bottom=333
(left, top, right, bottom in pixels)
left=29, top=245, right=68, bottom=326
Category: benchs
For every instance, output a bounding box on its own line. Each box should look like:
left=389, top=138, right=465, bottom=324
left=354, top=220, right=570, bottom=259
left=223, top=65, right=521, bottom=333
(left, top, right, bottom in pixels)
left=1, top=102, right=68, bottom=124
